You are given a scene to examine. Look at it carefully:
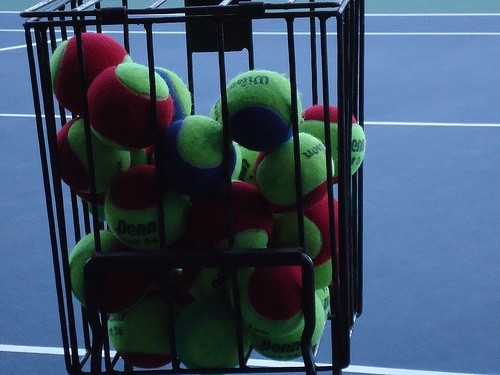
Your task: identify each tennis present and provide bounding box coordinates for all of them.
[50,30,366,368]
